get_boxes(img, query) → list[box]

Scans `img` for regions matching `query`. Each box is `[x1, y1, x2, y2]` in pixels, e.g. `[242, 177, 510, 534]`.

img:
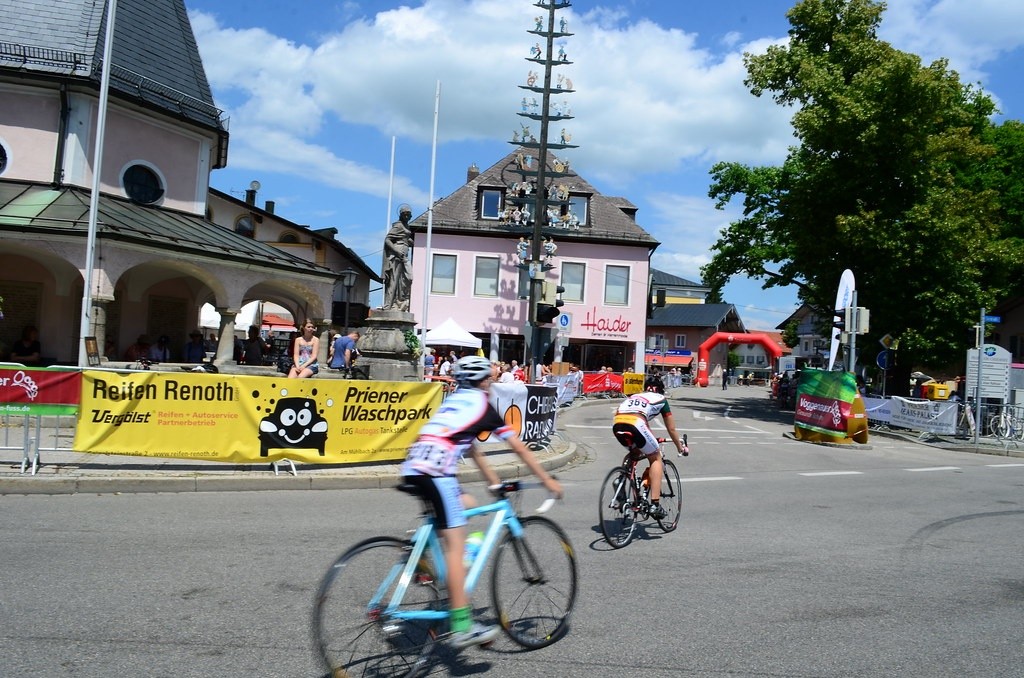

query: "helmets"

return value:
[644, 377, 664, 395]
[450, 355, 493, 381]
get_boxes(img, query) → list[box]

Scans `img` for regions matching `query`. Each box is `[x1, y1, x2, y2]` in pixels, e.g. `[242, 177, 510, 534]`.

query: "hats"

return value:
[189, 330, 203, 340]
[137, 334, 153, 346]
[159, 335, 169, 344]
[250, 325, 259, 331]
[334, 334, 342, 339]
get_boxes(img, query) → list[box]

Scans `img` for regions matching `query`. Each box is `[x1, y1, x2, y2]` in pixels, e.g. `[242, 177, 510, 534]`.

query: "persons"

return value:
[104, 335, 118, 361]
[183, 330, 243, 365]
[855, 375, 866, 397]
[598, 366, 613, 374]
[327, 330, 360, 379]
[425, 349, 579, 382]
[770, 372, 799, 411]
[379, 206, 415, 312]
[125, 334, 149, 363]
[266, 330, 275, 356]
[498, 0, 581, 264]
[288, 322, 320, 378]
[243, 326, 268, 365]
[612, 377, 684, 516]
[149, 335, 175, 363]
[10, 326, 41, 366]
[645, 368, 682, 389]
[722, 369, 727, 390]
[398, 355, 565, 649]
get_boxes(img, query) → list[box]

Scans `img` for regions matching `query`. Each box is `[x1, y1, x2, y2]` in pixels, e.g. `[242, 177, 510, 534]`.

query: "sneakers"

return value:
[404, 554, 439, 583]
[448, 621, 502, 649]
[648, 503, 668, 516]
[612, 478, 625, 502]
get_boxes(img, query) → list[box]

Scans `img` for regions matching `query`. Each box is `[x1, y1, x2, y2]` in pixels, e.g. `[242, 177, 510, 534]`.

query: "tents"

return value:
[415, 317, 482, 358]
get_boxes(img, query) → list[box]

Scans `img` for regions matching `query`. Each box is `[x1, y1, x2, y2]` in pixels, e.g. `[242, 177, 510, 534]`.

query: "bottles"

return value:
[465, 529, 484, 570]
[641, 467, 650, 487]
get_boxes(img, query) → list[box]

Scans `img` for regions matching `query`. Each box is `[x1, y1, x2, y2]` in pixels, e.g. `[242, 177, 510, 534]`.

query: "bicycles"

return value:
[957, 401, 998, 438]
[990, 403, 1024, 441]
[311, 474, 579, 678]
[599, 431, 687, 548]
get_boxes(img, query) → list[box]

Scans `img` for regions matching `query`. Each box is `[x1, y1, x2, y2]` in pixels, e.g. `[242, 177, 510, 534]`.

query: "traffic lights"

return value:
[537, 303, 560, 323]
[545, 282, 565, 307]
[833, 308, 850, 332]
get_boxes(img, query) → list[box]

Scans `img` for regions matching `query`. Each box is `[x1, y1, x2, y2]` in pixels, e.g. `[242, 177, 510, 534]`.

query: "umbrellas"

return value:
[262, 313, 294, 331]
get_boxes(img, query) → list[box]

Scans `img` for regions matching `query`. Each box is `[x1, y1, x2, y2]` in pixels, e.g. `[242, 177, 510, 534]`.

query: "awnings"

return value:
[645, 355, 694, 367]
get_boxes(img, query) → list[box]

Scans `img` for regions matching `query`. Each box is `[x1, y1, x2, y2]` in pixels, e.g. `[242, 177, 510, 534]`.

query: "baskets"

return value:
[353, 364, 370, 379]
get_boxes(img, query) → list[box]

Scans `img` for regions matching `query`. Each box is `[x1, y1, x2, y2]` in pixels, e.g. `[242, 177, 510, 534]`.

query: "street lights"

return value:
[340, 267, 359, 336]
